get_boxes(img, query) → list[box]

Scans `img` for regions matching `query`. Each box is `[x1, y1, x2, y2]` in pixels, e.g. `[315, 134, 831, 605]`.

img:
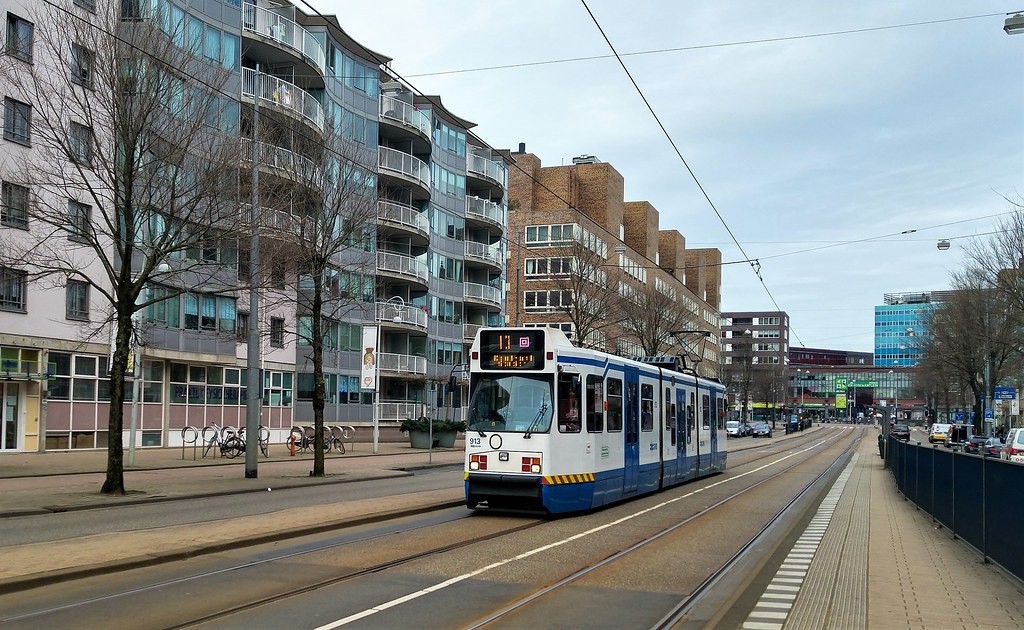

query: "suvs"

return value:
[999, 428, 1024, 463]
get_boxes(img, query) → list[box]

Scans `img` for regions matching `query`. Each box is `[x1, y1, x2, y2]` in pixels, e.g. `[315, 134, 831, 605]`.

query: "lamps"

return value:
[743, 328, 752, 334]
[936, 239, 951, 251]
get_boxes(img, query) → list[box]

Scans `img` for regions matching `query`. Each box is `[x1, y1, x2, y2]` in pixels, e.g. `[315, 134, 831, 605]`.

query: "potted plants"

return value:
[400, 416, 467, 448]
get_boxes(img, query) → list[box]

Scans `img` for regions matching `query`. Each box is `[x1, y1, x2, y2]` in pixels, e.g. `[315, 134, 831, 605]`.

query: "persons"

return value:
[995, 428, 998, 438]
[1000, 423, 1005, 443]
[858, 417, 861, 424]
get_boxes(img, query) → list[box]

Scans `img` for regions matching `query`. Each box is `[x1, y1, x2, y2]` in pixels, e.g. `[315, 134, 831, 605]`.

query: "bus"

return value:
[448, 326, 729, 515]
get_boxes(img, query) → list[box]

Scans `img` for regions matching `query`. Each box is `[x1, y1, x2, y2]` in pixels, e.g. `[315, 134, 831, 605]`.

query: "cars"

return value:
[726, 421, 753, 436]
[891, 424, 911, 442]
[961, 435, 1006, 458]
[928, 423, 953, 443]
[783, 415, 798, 430]
[751, 423, 772, 438]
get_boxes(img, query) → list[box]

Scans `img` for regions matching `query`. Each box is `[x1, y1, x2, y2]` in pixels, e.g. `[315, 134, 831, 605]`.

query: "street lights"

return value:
[797, 369, 810, 405]
[889, 360, 898, 408]
[726, 385, 735, 420]
[127, 232, 174, 468]
[752, 391, 759, 421]
[373, 296, 404, 453]
[776, 382, 784, 407]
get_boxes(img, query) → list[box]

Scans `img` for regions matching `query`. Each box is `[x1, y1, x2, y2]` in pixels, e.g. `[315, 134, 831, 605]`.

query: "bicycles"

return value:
[286, 426, 346, 454]
[203, 421, 271, 459]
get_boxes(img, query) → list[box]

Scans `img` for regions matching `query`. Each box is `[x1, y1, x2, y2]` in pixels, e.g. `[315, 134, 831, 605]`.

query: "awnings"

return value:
[788, 402, 825, 410]
[753, 402, 774, 408]
